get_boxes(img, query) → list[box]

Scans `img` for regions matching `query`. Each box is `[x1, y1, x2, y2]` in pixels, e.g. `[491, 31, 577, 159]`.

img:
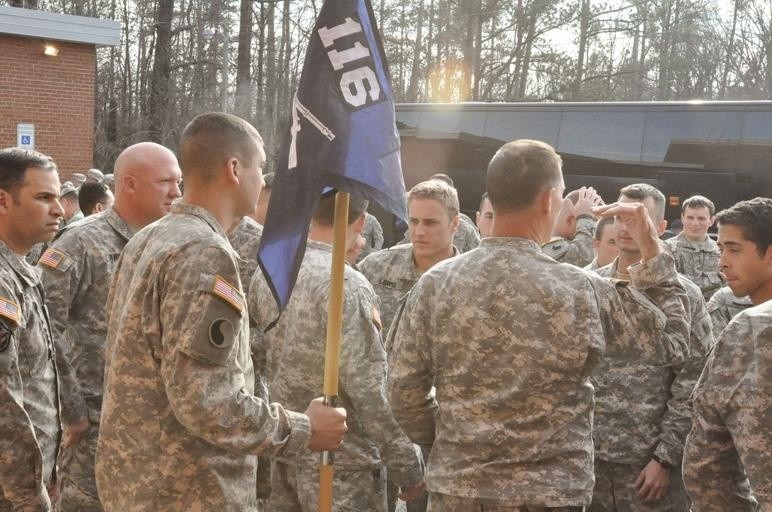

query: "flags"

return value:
[252, 1, 410, 333]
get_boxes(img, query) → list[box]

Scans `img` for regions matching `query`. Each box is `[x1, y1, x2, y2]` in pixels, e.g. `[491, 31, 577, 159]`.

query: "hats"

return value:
[60, 169, 115, 200]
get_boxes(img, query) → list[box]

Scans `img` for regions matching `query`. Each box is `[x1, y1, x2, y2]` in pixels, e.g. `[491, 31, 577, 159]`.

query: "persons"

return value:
[1, 109, 771, 511]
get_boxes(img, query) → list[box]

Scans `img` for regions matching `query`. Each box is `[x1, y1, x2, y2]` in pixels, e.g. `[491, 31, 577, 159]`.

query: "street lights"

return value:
[203, 27, 231, 114]
